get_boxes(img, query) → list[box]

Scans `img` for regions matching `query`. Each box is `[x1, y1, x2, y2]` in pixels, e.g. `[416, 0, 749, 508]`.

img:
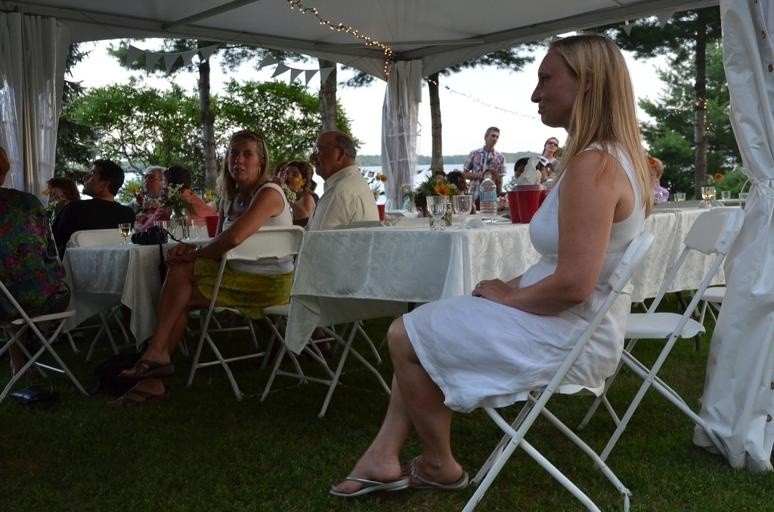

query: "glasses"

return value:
[548, 141, 558, 148]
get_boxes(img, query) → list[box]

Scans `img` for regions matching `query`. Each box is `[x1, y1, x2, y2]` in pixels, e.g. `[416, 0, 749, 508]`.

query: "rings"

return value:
[476, 283, 483, 288]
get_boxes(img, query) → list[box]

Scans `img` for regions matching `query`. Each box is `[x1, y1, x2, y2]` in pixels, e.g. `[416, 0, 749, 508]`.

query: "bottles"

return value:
[479, 173, 497, 223]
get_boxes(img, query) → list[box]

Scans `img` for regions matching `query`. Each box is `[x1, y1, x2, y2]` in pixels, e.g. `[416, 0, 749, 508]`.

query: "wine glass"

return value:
[190, 219, 203, 241]
[427, 196, 446, 229]
[118, 223, 131, 247]
[452, 195, 474, 230]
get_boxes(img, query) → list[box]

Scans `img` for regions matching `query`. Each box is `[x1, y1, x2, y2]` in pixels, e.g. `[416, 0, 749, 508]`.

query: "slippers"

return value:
[403, 454, 468, 490]
[119, 360, 175, 378]
[106, 387, 169, 408]
[330, 477, 409, 497]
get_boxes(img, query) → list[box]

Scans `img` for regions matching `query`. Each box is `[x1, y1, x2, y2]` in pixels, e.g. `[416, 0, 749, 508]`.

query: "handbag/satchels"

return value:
[96, 336, 152, 395]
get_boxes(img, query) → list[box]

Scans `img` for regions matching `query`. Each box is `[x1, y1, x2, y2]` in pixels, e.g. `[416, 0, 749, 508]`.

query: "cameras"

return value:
[131, 225, 169, 245]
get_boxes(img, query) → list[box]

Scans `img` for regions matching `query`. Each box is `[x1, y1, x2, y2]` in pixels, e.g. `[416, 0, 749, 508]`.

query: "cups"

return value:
[721, 191, 731, 200]
[700, 186, 716, 201]
[153, 220, 162, 227]
[205, 216, 220, 238]
[674, 192, 686, 201]
[162, 220, 176, 238]
[507, 185, 551, 223]
[738, 193, 751, 210]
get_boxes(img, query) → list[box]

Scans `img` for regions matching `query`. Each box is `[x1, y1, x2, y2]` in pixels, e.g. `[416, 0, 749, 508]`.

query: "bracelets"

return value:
[196, 245, 202, 257]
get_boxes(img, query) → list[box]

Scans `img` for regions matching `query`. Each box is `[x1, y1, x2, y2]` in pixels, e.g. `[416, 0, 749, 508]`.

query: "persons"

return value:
[304, 131, 380, 354]
[330, 34, 652, 498]
[0, 146, 71, 379]
[414, 127, 561, 217]
[107, 129, 294, 410]
[127, 165, 172, 224]
[51, 159, 136, 261]
[651, 157, 670, 204]
[47, 176, 81, 206]
[134, 166, 218, 232]
[275, 163, 288, 179]
[284, 161, 316, 227]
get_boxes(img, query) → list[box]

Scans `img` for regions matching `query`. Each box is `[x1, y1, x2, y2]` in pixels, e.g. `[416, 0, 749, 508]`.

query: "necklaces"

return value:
[236, 193, 253, 207]
